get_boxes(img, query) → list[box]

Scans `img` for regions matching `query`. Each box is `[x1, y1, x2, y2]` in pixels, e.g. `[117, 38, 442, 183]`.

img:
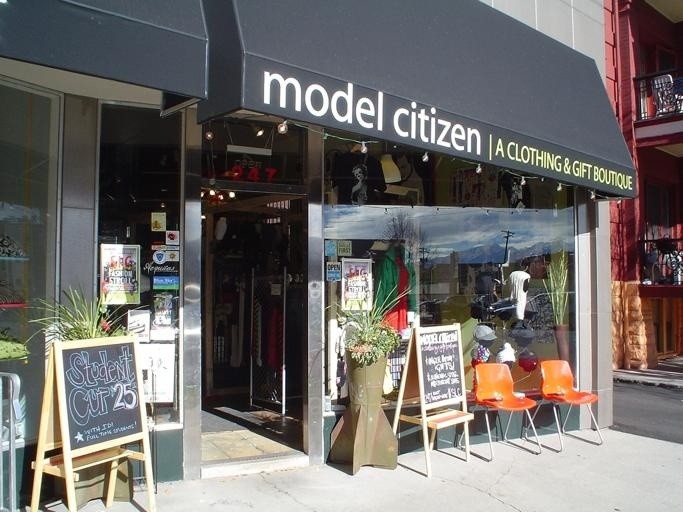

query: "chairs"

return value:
[460, 362, 542, 463]
[524, 359, 604, 453]
[650, 74, 683, 118]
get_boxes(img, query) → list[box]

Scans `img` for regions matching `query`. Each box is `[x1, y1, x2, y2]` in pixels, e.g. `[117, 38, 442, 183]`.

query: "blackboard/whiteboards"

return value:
[415, 323, 466, 410]
[53, 334, 148, 458]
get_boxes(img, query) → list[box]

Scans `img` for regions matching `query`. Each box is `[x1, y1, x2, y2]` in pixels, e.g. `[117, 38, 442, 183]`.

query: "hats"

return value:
[509, 319, 534, 338]
[519, 349, 536, 363]
[471, 345, 490, 368]
[496, 343, 517, 364]
[473, 324, 498, 342]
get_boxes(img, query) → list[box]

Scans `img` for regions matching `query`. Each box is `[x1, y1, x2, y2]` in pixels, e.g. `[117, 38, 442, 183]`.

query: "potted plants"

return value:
[26, 283, 149, 511]
[541, 252, 570, 361]
[331, 282, 409, 475]
